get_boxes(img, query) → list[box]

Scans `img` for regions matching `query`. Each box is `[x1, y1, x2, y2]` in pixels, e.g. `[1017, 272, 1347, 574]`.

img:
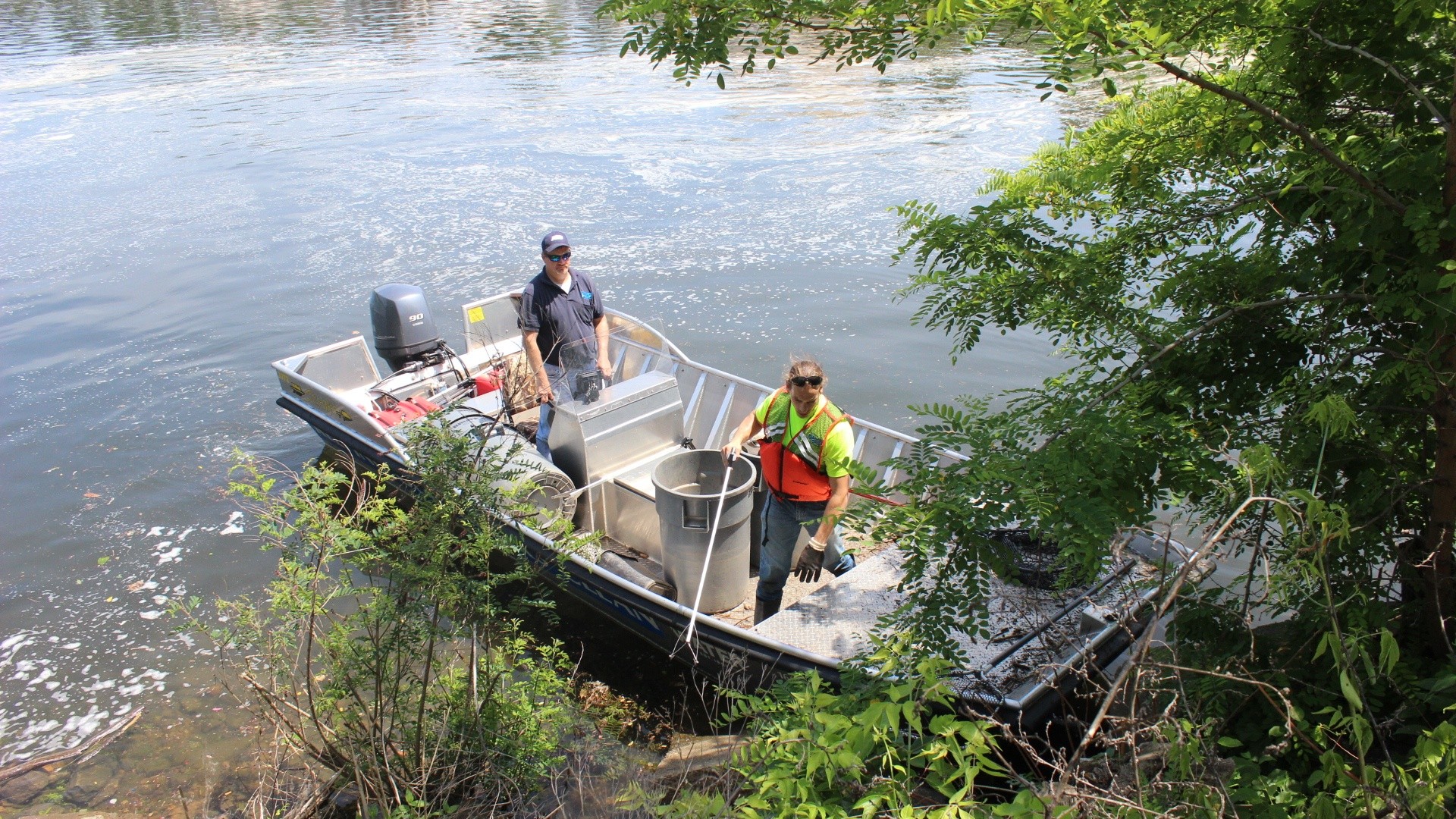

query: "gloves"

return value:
[794, 536, 827, 583]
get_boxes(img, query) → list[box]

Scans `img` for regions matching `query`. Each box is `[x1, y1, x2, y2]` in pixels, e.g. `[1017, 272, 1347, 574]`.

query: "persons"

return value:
[516, 231, 612, 464]
[720, 351, 858, 626]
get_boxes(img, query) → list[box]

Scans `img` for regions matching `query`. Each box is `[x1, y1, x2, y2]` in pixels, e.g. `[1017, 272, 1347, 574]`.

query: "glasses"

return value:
[789, 376, 823, 387]
[544, 251, 571, 262]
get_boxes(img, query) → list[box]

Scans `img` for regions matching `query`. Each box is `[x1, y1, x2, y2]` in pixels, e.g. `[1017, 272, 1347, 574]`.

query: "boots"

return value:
[754, 594, 781, 626]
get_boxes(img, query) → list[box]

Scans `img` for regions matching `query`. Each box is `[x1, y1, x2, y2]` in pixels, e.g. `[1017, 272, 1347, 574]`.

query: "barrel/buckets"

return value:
[650, 448, 757, 613]
[729, 425, 810, 570]
[371, 396, 578, 541]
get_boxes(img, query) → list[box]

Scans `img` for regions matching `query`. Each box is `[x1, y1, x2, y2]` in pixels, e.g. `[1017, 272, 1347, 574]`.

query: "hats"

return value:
[541, 232, 571, 252]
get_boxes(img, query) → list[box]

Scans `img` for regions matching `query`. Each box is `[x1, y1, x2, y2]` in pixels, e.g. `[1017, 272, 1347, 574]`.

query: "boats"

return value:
[272, 282, 1218, 745]
